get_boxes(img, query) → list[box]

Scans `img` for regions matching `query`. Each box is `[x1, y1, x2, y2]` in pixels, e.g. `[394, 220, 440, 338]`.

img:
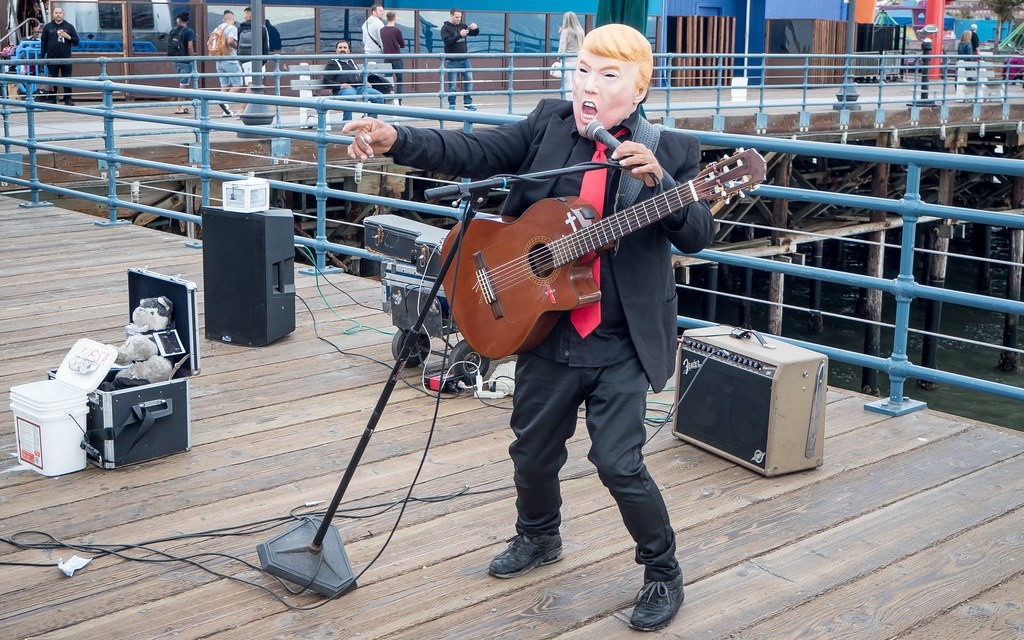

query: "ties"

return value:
[569, 127, 627, 339]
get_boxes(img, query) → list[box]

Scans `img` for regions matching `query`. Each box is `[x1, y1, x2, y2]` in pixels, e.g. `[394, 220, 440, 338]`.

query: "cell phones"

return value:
[466, 26, 471, 30]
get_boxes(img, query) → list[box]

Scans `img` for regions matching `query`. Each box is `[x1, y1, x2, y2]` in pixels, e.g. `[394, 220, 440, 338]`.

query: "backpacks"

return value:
[167, 27, 187, 56]
[237, 29, 252, 55]
[207, 26, 230, 56]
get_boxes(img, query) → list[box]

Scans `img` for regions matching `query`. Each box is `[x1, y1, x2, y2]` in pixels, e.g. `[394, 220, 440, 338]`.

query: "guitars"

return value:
[441, 146, 769, 358]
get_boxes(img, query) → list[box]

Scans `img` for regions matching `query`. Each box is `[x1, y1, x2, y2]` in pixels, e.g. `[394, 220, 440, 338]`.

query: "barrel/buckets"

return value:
[8, 382, 99, 475]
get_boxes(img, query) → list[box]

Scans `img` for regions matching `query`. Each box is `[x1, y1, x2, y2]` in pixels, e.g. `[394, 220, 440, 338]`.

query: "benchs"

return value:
[956, 59, 1006, 104]
[288, 61, 401, 133]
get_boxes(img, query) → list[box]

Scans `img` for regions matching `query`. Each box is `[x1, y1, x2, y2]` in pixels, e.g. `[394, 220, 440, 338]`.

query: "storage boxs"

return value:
[222, 179, 270, 214]
[46, 267, 201, 468]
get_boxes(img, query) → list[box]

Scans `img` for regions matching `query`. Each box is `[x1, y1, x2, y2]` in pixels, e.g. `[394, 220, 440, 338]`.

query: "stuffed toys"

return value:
[110, 294, 173, 365]
[113, 354, 171, 386]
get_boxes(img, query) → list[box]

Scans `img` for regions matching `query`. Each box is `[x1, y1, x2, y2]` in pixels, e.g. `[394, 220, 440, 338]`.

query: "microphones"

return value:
[584, 119, 646, 168]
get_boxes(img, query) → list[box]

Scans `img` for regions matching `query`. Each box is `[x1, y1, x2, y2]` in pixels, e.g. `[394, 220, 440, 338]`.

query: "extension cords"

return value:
[474, 390, 504, 399]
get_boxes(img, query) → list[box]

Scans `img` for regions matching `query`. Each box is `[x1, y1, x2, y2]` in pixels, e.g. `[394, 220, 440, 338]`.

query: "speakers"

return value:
[671, 326, 828, 477]
[201, 206, 296, 347]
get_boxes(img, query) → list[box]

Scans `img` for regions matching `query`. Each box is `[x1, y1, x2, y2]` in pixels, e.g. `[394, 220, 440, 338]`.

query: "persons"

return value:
[379, 11, 406, 106]
[341, 25, 715, 631]
[426, 25, 433, 54]
[41, 7, 79, 107]
[957, 31, 974, 81]
[213, 10, 244, 118]
[969, 24, 981, 69]
[236, 6, 269, 119]
[361, 4, 386, 77]
[265, 20, 282, 52]
[168, 12, 196, 114]
[556, 11, 585, 102]
[441, 7, 479, 111]
[323, 40, 386, 132]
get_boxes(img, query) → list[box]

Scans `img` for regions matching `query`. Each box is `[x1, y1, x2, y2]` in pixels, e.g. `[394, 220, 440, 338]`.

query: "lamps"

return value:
[916, 23, 939, 42]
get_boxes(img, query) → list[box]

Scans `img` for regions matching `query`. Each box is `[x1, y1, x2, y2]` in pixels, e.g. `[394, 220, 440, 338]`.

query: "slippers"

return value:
[175, 108, 189, 114]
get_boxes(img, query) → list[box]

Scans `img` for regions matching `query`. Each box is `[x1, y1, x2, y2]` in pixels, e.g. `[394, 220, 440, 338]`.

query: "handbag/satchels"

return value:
[550, 58, 568, 79]
[368, 75, 394, 93]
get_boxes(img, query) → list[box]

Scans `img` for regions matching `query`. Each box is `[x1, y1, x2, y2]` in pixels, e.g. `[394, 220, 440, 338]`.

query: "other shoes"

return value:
[223, 110, 233, 117]
[220, 104, 230, 114]
[64, 99, 75, 105]
[465, 105, 477, 110]
[448, 103, 456, 110]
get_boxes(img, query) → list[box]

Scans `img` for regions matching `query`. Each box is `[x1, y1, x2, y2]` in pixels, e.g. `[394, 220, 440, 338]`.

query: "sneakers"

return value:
[490, 532, 564, 579]
[629, 574, 684, 632]
